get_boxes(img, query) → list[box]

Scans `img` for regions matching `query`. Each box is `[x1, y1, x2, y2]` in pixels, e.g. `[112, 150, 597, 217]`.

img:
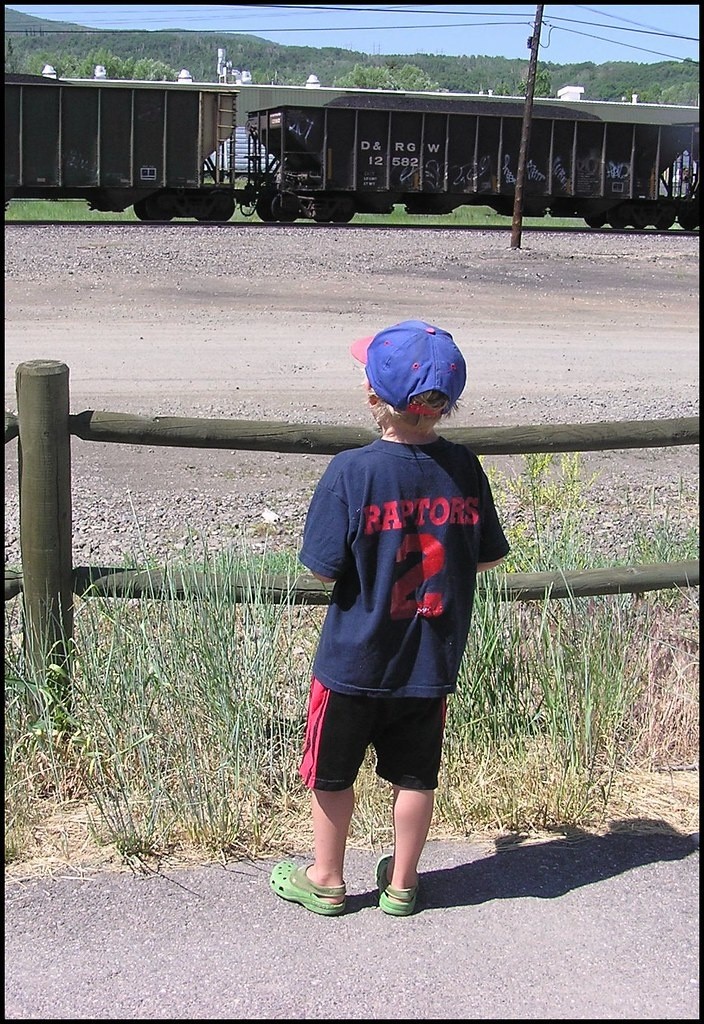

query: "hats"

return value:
[352, 320, 467, 415]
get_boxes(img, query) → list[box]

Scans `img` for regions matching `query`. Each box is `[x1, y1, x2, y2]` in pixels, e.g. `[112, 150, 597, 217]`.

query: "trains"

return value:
[3, 70, 699, 230]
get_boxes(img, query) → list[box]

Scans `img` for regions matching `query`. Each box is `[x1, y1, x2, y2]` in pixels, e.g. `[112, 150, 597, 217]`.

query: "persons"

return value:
[272, 322, 510, 915]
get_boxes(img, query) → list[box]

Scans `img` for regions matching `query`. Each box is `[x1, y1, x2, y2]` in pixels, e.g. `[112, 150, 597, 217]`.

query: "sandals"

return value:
[376, 852, 419, 915]
[270, 860, 347, 915]
